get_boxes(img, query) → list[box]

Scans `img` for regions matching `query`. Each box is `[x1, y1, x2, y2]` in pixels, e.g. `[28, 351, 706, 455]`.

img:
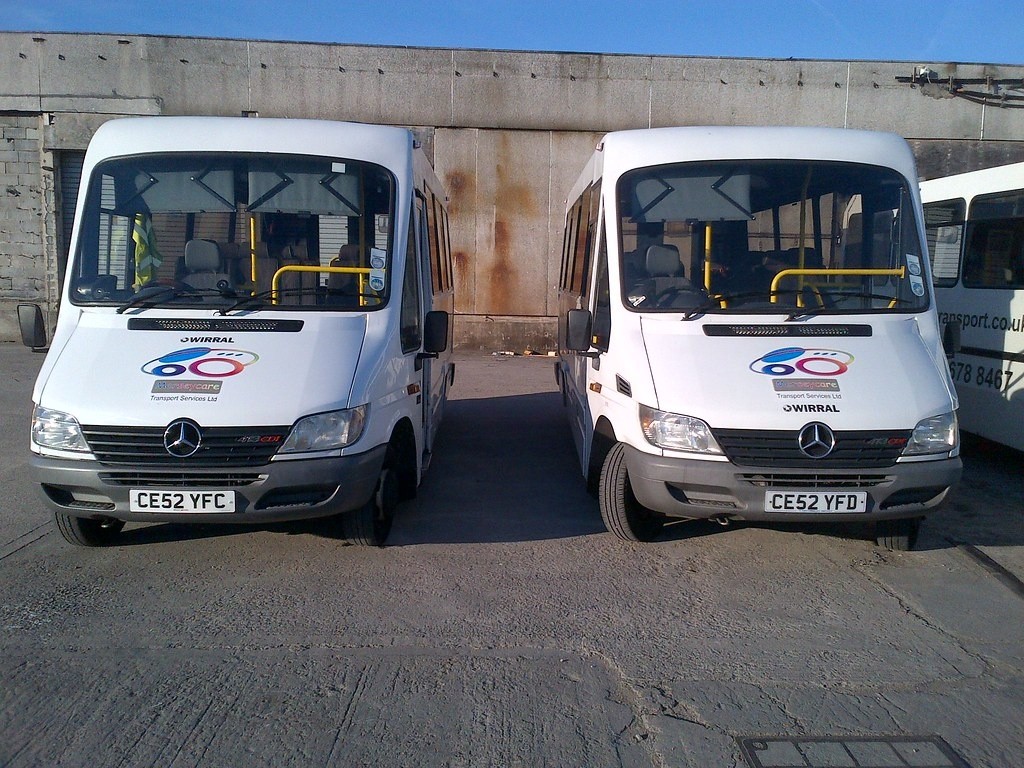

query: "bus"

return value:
[844, 161, 1023, 455]
[18, 115, 455, 546]
[551, 124, 963, 551]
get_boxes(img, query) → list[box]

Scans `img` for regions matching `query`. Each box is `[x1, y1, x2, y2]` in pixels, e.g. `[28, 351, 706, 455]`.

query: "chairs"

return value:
[727, 248, 827, 294]
[627, 244, 706, 307]
[325, 245, 370, 306]
[173, 238, 320, 304]
